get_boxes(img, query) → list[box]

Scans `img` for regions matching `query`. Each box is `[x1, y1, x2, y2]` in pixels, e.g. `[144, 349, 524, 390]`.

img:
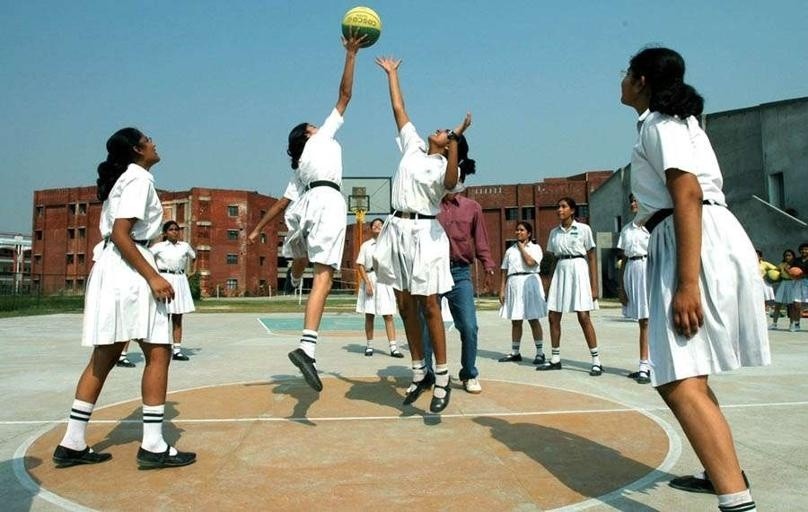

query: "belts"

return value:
[305, 181, 342, 193]
[627, 252, 648, 262]
[157, 268, 186, 276]
[104, 233, 153, 249]
[559, 252, 584, 259]
[642, 200, 718, 234]
[391, 206, 437, 221]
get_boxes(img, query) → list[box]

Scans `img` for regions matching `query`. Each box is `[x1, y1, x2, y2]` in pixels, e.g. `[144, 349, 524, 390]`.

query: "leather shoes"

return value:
[499, 353, 521, 362]
[628, 371, 650, 383]
[117, 357, 137, 368]
[289, 348, 322, 392]
[404, 370, 434, 405]
[534, 354, 546, 364]
[172, 350, 190, 361]
[669, 470, 749, 494]
[52, 444, 112, 464]
[590, 365, 602, 376]
[391, 350, 404, 358]
[137, 445, 197, 467]
[365, 346, 373, 356]
[430, 376, 450, 413]
[537, 361, 561, 370]
[285, 266, 298, 293]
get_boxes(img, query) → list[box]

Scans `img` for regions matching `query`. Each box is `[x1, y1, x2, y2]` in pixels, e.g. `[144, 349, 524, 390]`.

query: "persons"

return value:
[88, 240, 136, 369]
[245, 25, 373, 392]
[497, 221, 547, 363]
[755, 242, 807, 333]
[536, 197, 606, 375]
[354, 217, 405, 359]
[50, 127, 197, 469]
[617, 46, 776, 509]
[374, 51, 472, 412]
[147, 218, 198, 362]
[616, 192, 652, 381]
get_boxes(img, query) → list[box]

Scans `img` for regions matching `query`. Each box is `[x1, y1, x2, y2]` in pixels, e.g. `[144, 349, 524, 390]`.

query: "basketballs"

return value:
[341, 6, 383, 49]
[788, 266, 802, 276]
[767, 270, 780, 282]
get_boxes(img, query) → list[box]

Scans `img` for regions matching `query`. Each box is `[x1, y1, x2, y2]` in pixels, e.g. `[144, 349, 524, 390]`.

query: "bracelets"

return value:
[447, 130, 464, 144]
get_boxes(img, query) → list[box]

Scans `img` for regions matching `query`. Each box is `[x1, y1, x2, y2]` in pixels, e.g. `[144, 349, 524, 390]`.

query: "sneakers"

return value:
[788, 324, 800, 331]
[768, 323, 777, 329]
[462, 376, 482, 393]
